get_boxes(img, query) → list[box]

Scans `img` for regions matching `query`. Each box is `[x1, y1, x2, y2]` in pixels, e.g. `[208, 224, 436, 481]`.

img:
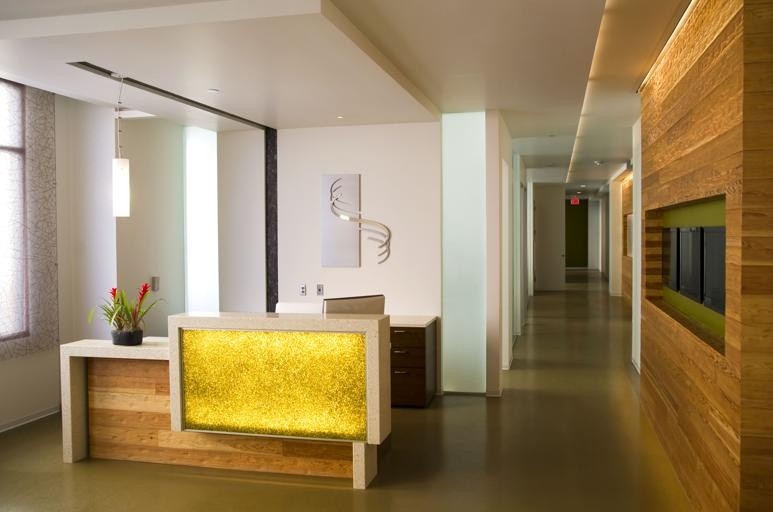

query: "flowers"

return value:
[84, 283, 167, 329]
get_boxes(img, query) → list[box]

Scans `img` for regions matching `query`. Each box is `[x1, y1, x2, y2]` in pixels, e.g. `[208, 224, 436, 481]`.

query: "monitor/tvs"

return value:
[322, 294, 385, 314]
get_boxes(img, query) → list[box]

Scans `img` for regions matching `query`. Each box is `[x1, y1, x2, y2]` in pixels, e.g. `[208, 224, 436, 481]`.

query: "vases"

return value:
[112, 329, 143, 346]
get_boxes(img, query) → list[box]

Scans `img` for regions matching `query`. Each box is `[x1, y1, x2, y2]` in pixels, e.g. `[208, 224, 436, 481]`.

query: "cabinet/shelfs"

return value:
[390, 327, 425, 409]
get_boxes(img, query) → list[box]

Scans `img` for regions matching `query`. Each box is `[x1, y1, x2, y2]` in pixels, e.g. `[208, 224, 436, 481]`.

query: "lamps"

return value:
[111, 72, 130, 218]
